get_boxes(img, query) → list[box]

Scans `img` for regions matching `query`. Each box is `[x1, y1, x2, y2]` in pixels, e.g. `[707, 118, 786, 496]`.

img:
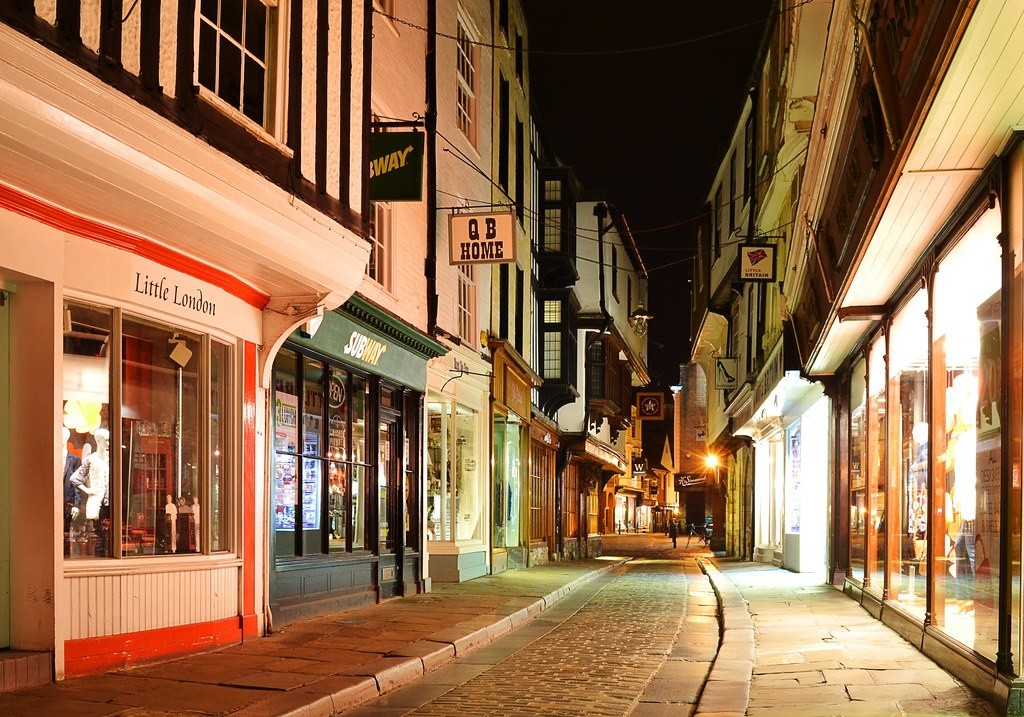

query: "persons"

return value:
[69, 429, 109, 557]
[178, 497, 192, 513]
[664, 519, 668, 535]
[669, 518, 676, 548]
[62, 427, 82, 561]
[165, 494, 177, 553]
[191, 497, 200, 552]
[677, 520, 681, 535]
[908, 373, 976, 617]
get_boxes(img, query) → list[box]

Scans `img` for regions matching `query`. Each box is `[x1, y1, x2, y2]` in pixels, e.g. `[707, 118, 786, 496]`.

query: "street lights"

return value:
[705, 452, 729, 558]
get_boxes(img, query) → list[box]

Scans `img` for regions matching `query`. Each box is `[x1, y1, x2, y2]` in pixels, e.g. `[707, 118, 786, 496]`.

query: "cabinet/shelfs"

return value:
[428, 413, 463, 544]
[272, 420, 364, 547]
[122, 434, 175, 555]
